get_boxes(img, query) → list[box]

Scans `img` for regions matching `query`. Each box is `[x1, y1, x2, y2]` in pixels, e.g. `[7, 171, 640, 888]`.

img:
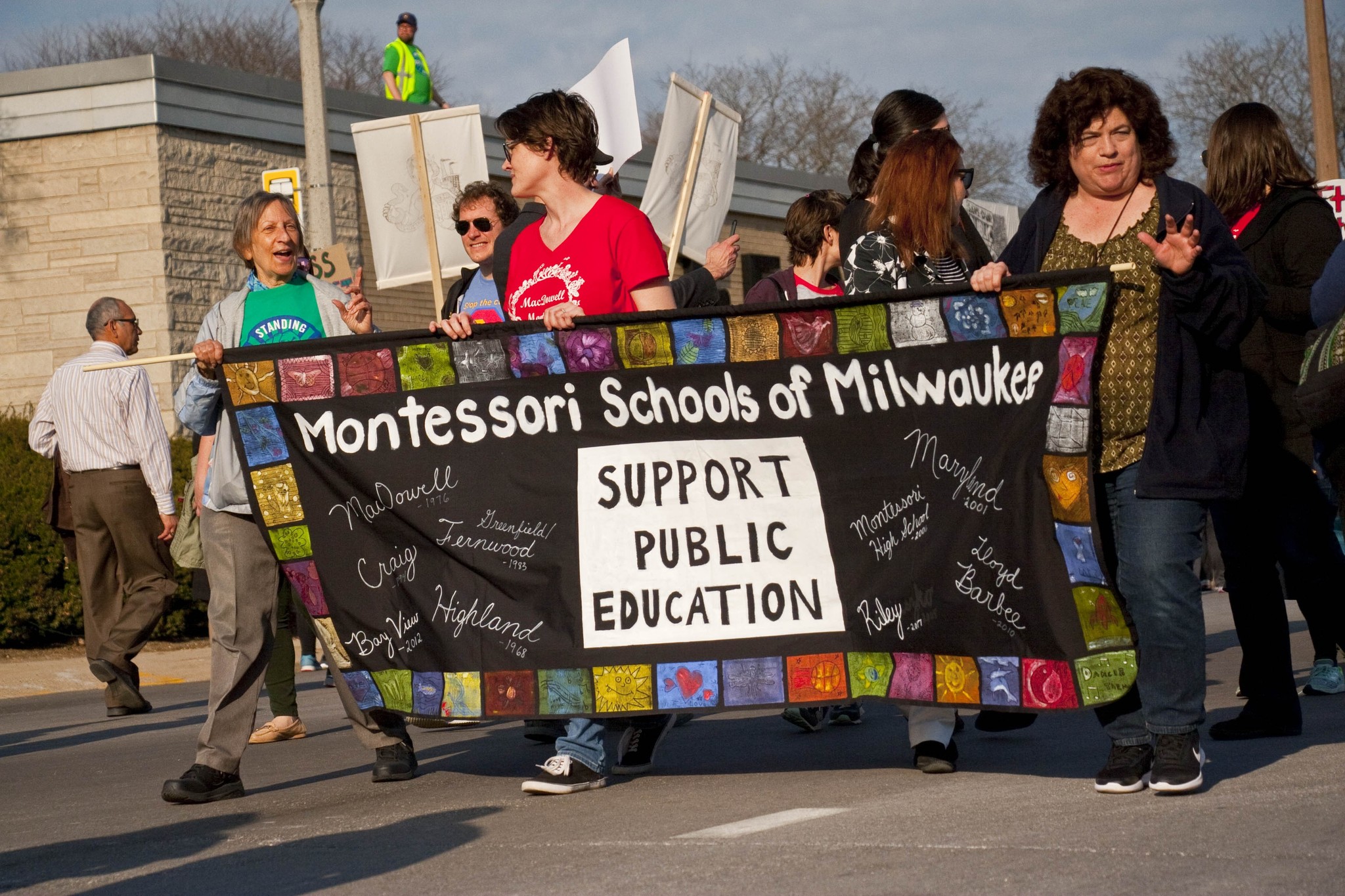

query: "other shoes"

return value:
[406, 715, 480, 728]
[250, 717, 307, 743]
[323, 672, 336, 688]
[319, 656, 329, 669]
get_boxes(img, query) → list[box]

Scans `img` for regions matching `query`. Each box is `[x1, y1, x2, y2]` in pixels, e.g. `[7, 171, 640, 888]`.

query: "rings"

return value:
[169, 532, 175, 536]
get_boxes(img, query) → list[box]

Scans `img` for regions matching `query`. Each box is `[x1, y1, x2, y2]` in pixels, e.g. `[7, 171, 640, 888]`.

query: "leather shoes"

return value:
[89, 660, 152, 717]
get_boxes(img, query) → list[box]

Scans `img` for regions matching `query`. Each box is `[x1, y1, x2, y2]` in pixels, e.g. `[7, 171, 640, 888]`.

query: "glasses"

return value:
[950, 167, 974, 190]
[502, 138, 525, 162]
[591, 168, 598, 182]
[399, 24, 414, 32]
[104, 318, 139, 330]
[296, 258, 312, 272]
[933, 124, 951, 132]
[455, 216, 502, 235]
[1200, 150, 1208, 169]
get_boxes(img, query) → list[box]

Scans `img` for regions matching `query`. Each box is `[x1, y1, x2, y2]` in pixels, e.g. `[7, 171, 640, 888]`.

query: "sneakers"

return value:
[161, 763, 244, 802]
[1201, 579, 1224, 592]
[522, 713, 694, 794]
[901, 660, 1344, 795]
[300, 655, 322, 671]
[372, 737, 415, 781]
[781, 702, 865, 729]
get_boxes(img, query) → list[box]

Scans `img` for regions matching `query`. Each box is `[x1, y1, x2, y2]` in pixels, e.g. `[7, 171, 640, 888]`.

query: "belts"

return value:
[104, 464, 141, 470]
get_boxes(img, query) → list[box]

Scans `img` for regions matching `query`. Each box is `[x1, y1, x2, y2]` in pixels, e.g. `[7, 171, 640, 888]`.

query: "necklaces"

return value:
[1095, 181, 1140, 260]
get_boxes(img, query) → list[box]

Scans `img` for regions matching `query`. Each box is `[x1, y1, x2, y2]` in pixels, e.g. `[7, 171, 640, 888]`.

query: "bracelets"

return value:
[440, 101, 445, 107]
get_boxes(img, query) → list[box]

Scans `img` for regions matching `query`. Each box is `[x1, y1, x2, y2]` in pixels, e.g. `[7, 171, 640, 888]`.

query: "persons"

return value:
[194, 434, 336, 742]
[28, 297, 177, 717]
[1204, 102, 1345, 737]
[430, 88, 675, 793]
[407, 180, 562, 734]
[746, 187, 862, 727]
[160, 189, 417, 802]
[972, 65, 1255, 792]
[591, 166, 743, 724]
[382, 12, 450, 108]
[840, 88, 1036, 771]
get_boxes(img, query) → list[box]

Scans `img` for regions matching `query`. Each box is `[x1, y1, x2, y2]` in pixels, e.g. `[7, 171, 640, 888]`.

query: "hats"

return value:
[592, 146, 613, 166]
[396, 12, 416, 29]
